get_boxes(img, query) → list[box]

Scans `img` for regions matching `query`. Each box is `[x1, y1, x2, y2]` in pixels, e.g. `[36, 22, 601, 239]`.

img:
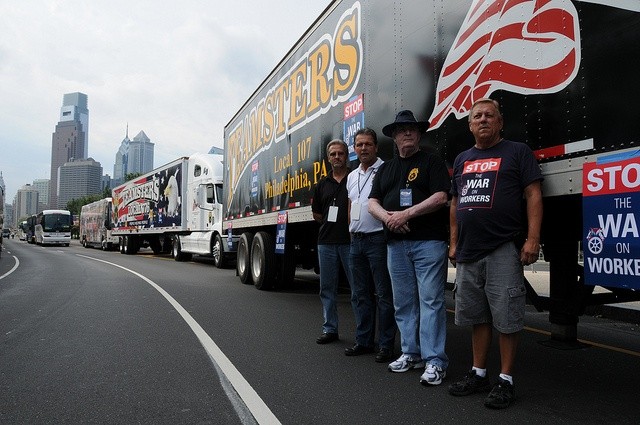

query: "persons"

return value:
[312, 139, 350, 345]
[344, 127, 398, 364]
[448, 98, 544, 410]
[368, 110, 452, 387]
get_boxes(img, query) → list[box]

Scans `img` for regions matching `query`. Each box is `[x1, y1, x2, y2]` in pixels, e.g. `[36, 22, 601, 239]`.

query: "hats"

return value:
[382, 110, 430, 137]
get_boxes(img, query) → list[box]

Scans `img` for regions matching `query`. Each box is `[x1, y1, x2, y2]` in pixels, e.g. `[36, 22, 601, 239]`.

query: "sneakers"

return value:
[448, 368, 489, 396]
[420, 362, 447, 385]
[484, 374, 516, 408]
[388, 353, 425, 372]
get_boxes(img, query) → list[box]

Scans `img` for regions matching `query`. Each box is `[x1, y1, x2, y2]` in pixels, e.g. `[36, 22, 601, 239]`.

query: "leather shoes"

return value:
[316, 332, 338, 343]
[374, 345, 393, 362]
[344, 344, 374, 355]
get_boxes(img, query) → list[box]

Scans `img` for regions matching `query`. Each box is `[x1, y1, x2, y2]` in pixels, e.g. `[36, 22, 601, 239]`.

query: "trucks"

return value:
[222, 0, 636, 290]
[112, 155, 224, 267]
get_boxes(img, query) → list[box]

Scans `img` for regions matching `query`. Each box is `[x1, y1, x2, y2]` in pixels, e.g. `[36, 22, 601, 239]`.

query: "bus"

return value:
[18, 221, 27, 241]
[27, 210, 70, 246]
[80, 198, 112, 250]
